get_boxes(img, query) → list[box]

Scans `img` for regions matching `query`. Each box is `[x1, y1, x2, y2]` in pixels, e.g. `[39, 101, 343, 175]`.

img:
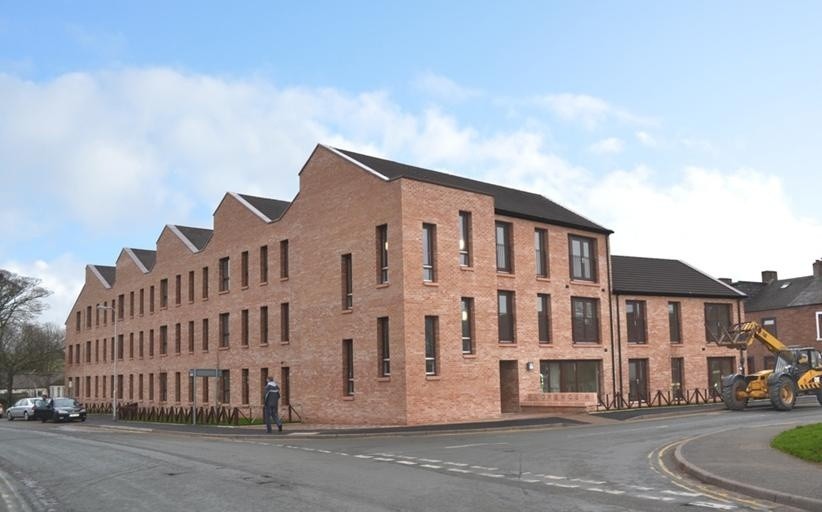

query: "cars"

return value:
[37, 397, 87, 422]
[6, 398, 42, 421]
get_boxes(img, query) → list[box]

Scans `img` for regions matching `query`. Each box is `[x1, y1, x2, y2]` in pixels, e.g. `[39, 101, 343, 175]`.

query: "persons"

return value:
[39, 395, 49, 423]
[264, 375, 282, 433]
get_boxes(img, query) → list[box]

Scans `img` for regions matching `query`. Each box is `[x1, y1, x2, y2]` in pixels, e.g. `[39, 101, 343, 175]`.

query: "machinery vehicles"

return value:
[704, 319, 822, 410]
